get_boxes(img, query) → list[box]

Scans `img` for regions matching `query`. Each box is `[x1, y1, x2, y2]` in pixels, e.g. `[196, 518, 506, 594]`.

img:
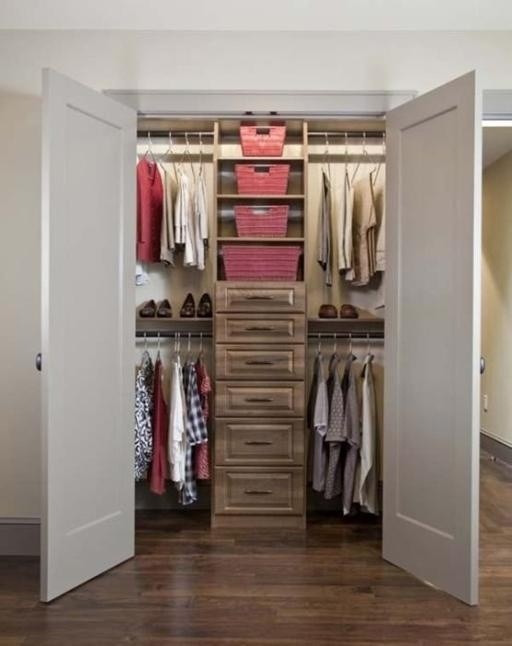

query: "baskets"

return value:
[220, 245, 303, 281]
[233, 205, 290, 237]
[234, 164, 292, 195]
[239, 125, 286, 157]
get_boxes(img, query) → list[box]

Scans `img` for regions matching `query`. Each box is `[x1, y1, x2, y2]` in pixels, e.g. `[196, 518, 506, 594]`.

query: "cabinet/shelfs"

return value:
[34, 63, 487, 614]
[211, 119, 307, 534]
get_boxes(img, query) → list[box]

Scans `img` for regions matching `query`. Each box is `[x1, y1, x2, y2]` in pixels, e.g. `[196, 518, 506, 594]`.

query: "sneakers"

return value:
[340, 303, 359, 319]
[318, 304, 337, 319]
[157, 299, 173, 318]
[139, 300, 157, 318]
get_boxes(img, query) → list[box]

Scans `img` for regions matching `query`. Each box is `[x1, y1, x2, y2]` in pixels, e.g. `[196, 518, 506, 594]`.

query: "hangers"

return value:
[317, 122, 386, 178]
[142, 128, 205, 180]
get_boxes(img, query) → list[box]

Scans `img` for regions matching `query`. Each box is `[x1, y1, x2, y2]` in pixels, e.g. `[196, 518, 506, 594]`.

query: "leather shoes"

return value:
[180, 293, 195, 317]
[197, 293, 212, 318]
